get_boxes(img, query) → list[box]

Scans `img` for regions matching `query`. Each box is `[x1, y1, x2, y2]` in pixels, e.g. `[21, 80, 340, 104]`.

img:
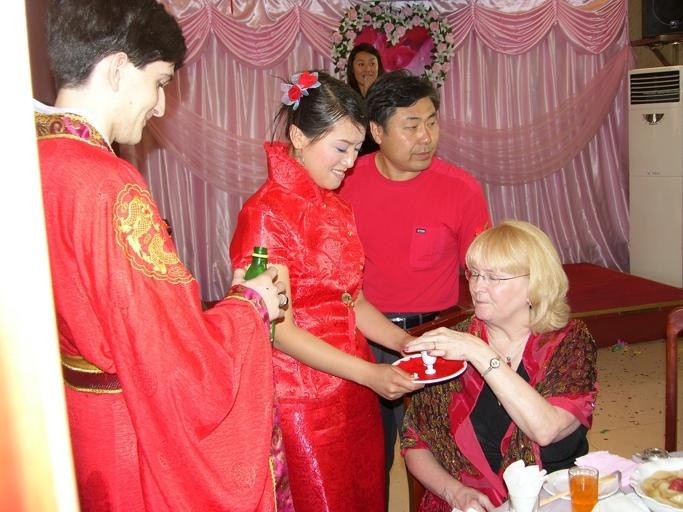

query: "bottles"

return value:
[240, 244, 276, 347]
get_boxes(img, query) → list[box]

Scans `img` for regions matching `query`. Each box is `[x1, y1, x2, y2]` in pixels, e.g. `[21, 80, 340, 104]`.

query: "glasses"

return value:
[464, 271, 530, 287]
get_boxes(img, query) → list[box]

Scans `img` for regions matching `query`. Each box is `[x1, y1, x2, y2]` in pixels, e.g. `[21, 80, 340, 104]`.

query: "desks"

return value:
[440, 262, 683, 452]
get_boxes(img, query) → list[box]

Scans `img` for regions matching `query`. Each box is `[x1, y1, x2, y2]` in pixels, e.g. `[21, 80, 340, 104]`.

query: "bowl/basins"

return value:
[630, 457, 682, 512]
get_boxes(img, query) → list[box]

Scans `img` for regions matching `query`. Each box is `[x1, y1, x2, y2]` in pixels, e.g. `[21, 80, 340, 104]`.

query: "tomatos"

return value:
[670, 477, 682, 492]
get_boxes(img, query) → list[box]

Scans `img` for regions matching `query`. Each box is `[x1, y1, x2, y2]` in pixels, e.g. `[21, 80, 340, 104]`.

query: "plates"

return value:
[542, 468, 619, 501]
[390, 353, 467, 384]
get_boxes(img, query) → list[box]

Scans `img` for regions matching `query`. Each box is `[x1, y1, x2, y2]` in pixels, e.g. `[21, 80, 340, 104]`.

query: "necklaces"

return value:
[487, 330, 530, 368]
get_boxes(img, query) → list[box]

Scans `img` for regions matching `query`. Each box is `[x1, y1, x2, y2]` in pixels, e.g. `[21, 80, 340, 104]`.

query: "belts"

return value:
[385, 311, 443, 330]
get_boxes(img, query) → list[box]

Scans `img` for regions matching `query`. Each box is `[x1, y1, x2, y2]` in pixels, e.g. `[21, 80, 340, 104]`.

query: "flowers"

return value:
[280, 70, 322, 111]
[332, 2, 455, 89]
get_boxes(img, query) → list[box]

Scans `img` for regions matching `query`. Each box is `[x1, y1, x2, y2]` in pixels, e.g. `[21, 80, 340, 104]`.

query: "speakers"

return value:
[641, 0, 683, 38]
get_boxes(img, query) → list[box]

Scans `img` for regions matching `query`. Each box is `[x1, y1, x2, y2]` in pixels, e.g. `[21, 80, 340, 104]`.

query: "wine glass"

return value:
[420, 351, 437, 377]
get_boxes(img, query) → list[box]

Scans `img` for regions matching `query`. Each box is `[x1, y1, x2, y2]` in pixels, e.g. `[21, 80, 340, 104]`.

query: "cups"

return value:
[507, 495, 539, 512]
[566, 465, 598, 512]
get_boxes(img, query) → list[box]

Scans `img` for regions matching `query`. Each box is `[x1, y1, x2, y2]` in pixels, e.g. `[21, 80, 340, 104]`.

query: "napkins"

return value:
[503, 459, 550, 512]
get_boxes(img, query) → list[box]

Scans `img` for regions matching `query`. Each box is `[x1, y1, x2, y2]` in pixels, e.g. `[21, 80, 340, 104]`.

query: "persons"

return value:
[228, 70, 419, 512]
[32, 1, 289, 512]
[346, 43, 384, 156]
[400, 217, 600, 512]
[334, 70, 492, 511]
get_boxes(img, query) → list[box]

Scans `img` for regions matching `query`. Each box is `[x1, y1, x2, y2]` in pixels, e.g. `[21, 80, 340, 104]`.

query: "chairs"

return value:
[403, 308, 475, 512]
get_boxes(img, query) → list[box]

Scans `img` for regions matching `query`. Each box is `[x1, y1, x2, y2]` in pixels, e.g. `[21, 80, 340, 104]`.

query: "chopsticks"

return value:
[540, 472, 615, 508]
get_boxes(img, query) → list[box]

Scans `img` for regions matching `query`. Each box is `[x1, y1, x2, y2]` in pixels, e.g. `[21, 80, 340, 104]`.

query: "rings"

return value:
[279, 293, 288, 308]
[433, 341, 435, 350]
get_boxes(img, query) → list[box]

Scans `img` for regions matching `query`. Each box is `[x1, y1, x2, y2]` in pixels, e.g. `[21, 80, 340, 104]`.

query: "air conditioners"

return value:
[627, 66, 683, 287]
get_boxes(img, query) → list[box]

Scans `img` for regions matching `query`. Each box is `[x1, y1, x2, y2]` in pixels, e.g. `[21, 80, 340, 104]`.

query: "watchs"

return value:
[480, 354, 501, 378]
